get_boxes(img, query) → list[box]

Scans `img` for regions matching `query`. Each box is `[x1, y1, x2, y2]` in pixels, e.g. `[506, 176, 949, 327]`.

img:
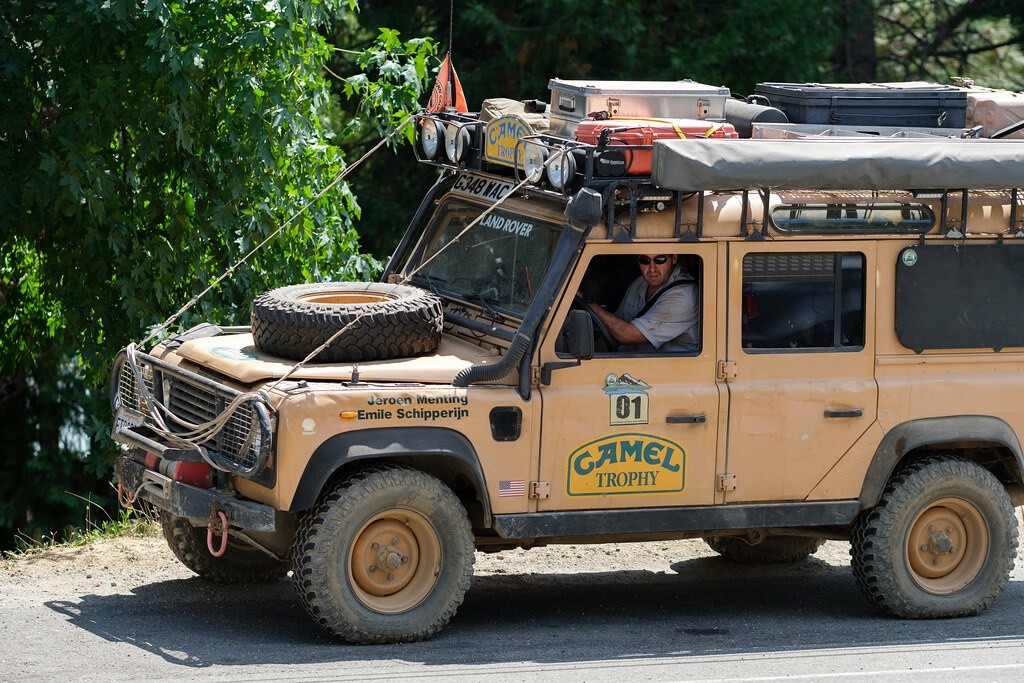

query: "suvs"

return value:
[108, 114, 1024, 647]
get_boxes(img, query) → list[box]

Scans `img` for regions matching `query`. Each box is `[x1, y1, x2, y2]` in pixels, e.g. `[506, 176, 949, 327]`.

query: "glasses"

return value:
[637, 254, 672, 265]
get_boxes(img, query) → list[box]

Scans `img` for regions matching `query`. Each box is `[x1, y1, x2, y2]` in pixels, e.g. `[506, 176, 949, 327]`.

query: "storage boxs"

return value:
[754, 81, 968, 129]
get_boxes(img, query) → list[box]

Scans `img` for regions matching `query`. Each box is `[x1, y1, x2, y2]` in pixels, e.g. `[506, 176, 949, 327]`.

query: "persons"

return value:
[576, 254, 702, 353]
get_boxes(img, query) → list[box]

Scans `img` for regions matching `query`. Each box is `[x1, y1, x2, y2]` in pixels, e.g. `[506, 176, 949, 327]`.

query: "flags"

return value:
[419, 53, 468, 127]
